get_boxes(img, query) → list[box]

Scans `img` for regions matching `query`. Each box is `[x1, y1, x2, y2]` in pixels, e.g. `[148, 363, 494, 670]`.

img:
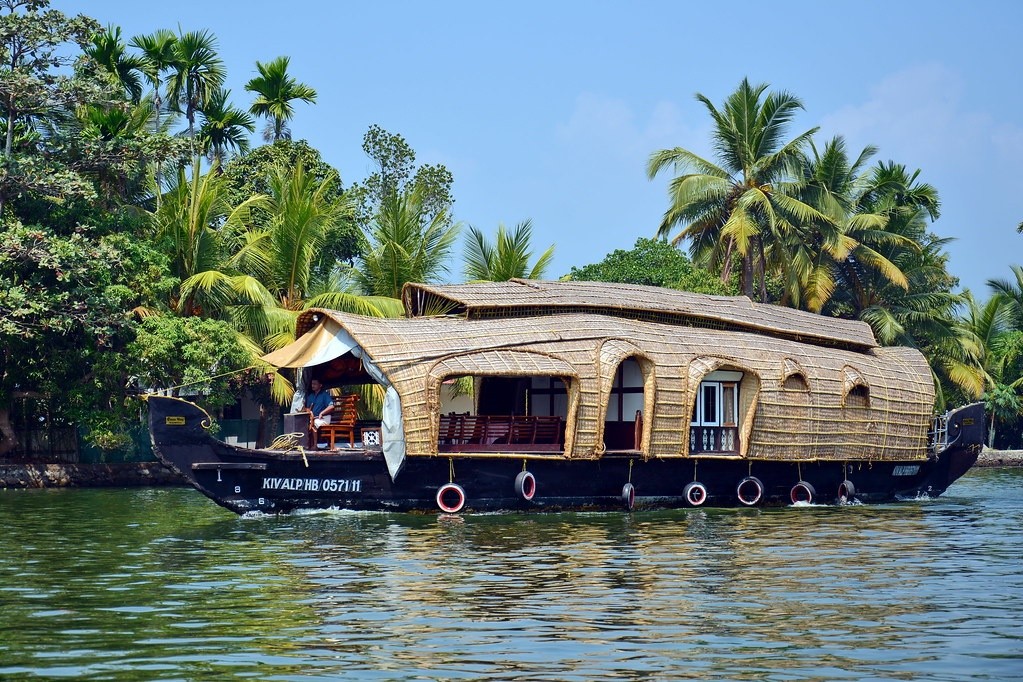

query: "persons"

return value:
[305, 377, 334, 451]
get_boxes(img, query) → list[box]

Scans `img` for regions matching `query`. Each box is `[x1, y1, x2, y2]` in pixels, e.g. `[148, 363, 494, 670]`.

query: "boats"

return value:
[147, 277, 987, 517]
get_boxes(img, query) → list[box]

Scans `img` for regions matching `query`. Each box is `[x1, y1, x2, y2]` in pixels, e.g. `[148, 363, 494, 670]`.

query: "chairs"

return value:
[439, 410, 563, 452]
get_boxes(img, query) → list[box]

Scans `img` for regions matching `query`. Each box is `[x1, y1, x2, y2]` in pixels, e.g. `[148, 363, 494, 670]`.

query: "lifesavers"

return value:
[682, 482, 707, 506]
[436, 483, 465, 514]
[790, 481, 816, 505]
[515, 470, 536, 501]
[621, 483, 634, 511]
[735, 476, 765, 506]
[838, 480, 855, 503]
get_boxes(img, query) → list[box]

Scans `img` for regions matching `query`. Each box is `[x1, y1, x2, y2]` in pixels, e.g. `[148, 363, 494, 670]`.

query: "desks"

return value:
[479, 423, 519, 445]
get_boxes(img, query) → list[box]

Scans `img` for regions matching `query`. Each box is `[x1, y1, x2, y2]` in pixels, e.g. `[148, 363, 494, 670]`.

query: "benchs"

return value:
[311, 394, 360, 451]
